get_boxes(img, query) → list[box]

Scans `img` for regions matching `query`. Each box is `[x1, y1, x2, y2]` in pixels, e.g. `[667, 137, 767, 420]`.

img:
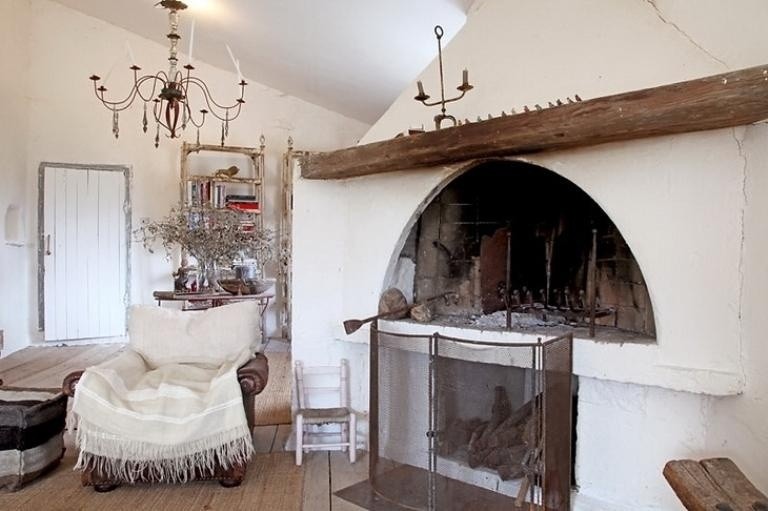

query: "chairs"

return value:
[61, 301, 267, 487]
[293, 360, 357, 466]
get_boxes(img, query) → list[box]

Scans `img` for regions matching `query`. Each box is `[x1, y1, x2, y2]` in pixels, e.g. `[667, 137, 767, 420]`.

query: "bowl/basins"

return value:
[218, 278, 277, 294]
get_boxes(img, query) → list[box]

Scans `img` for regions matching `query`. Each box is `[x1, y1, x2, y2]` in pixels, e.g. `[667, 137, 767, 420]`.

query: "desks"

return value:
[154, 288, 275, 343]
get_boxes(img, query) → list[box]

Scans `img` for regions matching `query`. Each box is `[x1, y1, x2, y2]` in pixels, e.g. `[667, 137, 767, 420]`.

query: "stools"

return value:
[663, 458, 768, 511]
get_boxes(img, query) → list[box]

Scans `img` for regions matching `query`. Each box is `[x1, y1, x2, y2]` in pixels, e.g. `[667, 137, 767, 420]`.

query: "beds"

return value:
[1, 346, 131, 493]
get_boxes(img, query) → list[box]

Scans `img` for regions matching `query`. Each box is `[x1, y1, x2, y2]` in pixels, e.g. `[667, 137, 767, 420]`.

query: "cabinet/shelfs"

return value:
[179, 141, 265, 279]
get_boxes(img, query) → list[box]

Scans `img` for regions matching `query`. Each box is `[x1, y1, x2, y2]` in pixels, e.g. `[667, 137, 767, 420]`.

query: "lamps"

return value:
[89, 0, 247, 153]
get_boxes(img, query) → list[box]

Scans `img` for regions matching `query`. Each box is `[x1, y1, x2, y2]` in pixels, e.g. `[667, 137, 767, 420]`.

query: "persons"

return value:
[172, 259, 197, 290]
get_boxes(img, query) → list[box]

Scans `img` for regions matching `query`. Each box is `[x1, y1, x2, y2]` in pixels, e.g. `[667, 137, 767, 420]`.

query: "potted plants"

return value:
[133, 202, 279, 297]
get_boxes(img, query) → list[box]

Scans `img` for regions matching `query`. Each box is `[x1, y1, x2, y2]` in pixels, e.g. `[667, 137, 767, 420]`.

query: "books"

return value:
[187, 179, 259, 234]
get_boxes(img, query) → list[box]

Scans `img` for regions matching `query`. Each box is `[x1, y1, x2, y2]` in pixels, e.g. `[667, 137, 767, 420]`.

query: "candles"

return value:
[463, 67, 468, 84]
[417, 81, 424, 96]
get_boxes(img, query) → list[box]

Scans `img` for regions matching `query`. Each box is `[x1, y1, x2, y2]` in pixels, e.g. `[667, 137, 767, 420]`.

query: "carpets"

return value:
[1, 344, 292, 427]
[1, 451, 330, 511]
